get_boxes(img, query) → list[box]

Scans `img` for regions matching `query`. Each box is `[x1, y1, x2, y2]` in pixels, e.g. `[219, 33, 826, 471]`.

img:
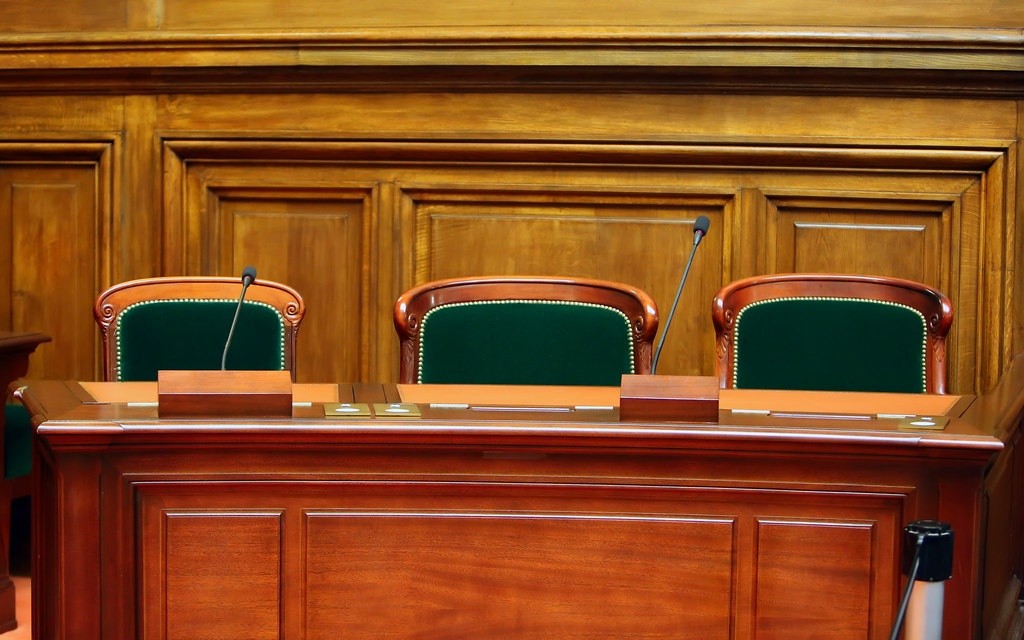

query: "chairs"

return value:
[394, 273, 661, 387]
[91, 276, 306, 380]
[711, 274, 955, 395]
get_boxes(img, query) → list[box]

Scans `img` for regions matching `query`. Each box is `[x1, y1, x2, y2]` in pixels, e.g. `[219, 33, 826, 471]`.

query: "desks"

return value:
[40, 403, 1005, 640]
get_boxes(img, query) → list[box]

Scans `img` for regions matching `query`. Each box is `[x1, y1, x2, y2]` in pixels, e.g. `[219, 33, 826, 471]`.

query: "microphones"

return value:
[220, 266, 258, 370]
[650, 216, 711, 375]
